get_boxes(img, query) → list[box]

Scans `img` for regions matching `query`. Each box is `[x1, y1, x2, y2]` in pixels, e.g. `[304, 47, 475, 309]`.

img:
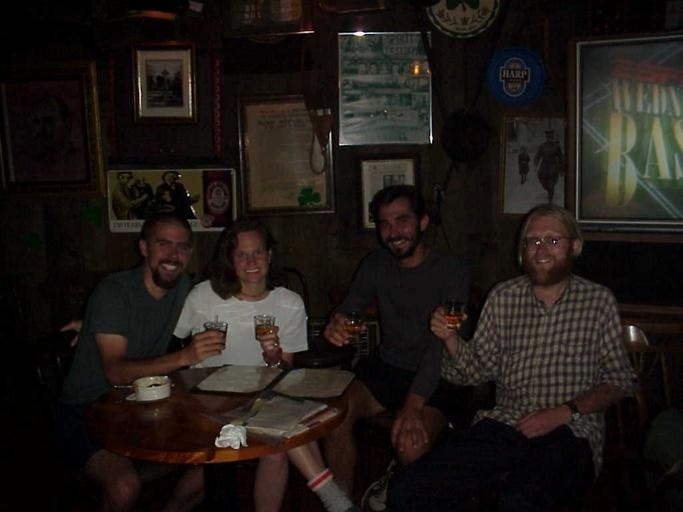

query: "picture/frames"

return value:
[105, 163, 242, 244]
[331, 28, 442, 153]
[355, 153, 421, 232]
[564, 31, 682, 247]
[231, 92, 336, 219]
[492, 107, 565, 223]
[0, 59, 106, 201]
[131, 40, 199, 128]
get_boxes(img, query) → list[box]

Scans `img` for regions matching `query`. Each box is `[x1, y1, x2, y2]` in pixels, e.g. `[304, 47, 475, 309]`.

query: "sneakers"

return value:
[361, 458, 396, 511]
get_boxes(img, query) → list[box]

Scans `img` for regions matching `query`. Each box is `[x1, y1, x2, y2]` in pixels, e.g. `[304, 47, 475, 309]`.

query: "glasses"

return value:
[521, 236, 567, 249]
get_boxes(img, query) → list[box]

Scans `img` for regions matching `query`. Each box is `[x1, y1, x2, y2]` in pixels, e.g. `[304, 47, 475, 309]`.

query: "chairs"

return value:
[615, 317, 682, 451]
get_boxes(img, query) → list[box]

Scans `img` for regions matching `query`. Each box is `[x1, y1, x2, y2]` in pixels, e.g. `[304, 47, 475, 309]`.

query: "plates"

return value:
[192, 365, 285, 397]
[273, 368, 358, 400]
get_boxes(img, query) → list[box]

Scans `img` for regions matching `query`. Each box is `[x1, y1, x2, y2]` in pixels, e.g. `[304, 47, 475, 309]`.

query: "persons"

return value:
[113, 170, 195, 216]
[535, 128, 564, 204]
[53, 211, 224, 506]
[360, 205, 641, 507]
[513, 142, 533, 186]
[60, 218, 353, 506]
[324, 184, 465, 464]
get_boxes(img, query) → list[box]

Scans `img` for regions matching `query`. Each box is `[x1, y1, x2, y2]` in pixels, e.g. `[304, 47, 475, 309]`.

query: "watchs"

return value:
[261, 359, 286, 368]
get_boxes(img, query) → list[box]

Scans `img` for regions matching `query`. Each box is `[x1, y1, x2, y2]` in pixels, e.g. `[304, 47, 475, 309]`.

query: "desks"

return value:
[84, 370, 348, 511]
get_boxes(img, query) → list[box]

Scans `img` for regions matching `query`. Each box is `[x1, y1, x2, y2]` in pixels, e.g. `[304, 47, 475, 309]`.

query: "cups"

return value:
[439, 296, 467, 329]
[253, 314, 274, 343]
[132, 375, 171, 402]
[203, 320, 228, 349]
[342, 304, 364, 333]
